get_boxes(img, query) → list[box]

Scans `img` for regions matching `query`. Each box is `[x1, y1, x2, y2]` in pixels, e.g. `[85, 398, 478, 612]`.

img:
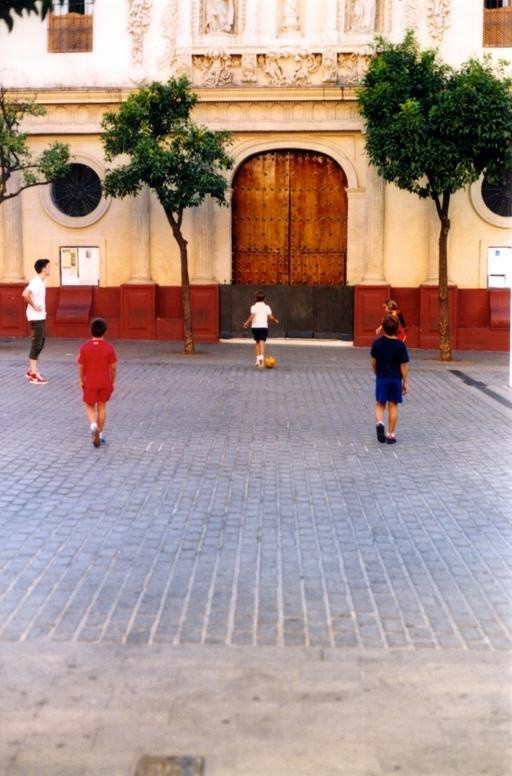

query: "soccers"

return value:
[265, 354, 276, 368]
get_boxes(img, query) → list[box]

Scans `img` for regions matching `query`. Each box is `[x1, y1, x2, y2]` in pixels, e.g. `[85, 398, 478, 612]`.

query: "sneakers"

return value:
[25, 370, 48, 384]
[92, 424, 105, 446]
[376, 423, 386, 443]
[387, 433, 397, 443]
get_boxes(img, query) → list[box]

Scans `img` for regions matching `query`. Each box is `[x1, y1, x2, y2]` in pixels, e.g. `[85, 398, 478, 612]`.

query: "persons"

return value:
[370, 314, 409, 444]
[79, 320, 116, 447]
[22, 260, 53, 385]
[243, 291, 279, 370]
[376, 300, 409, 349]
